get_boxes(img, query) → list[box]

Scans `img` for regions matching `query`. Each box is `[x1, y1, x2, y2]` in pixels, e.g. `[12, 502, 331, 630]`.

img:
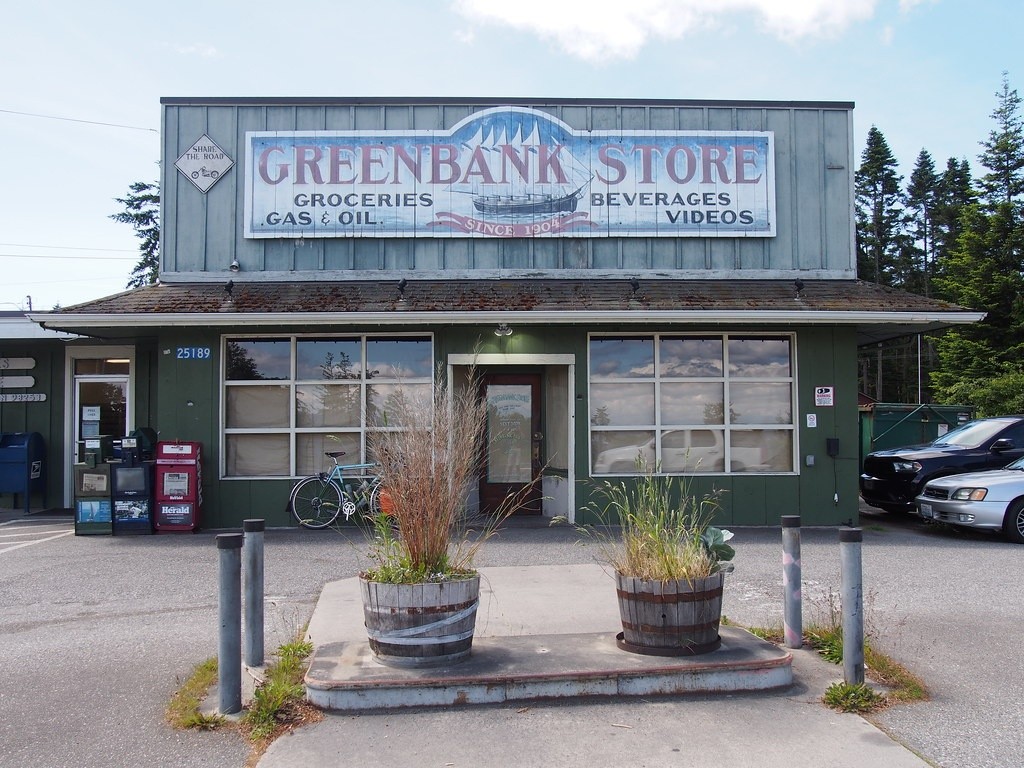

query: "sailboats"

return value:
[444, 120, 595, 215]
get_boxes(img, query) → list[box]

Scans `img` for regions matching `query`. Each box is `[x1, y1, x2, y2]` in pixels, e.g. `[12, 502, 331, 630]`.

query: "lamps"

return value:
[629, 276, 639, 294]
[395, 277, 409, 300]
[228, 259, 242, 273]
[495, 324, 514, 337]
[794, 277, 805, 299]
[223, 280, 236, 300]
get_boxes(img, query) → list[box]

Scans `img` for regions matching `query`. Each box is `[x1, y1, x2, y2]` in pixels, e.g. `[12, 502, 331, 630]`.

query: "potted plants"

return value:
[327, 334, 547, 669]
[544, 446, 737, 660]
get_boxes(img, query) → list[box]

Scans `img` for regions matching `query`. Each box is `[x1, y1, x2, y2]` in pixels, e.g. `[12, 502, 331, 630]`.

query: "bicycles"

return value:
[288, 449, 435, 532]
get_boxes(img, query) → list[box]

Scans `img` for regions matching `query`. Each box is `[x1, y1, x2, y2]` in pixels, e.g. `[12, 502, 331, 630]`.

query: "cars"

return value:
[861, 412, 1024, 509]
[913, 454, 1024, 545]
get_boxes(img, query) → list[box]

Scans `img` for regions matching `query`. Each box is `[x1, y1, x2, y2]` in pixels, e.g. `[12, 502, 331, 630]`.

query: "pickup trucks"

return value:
[594, 427, 763, 474]
